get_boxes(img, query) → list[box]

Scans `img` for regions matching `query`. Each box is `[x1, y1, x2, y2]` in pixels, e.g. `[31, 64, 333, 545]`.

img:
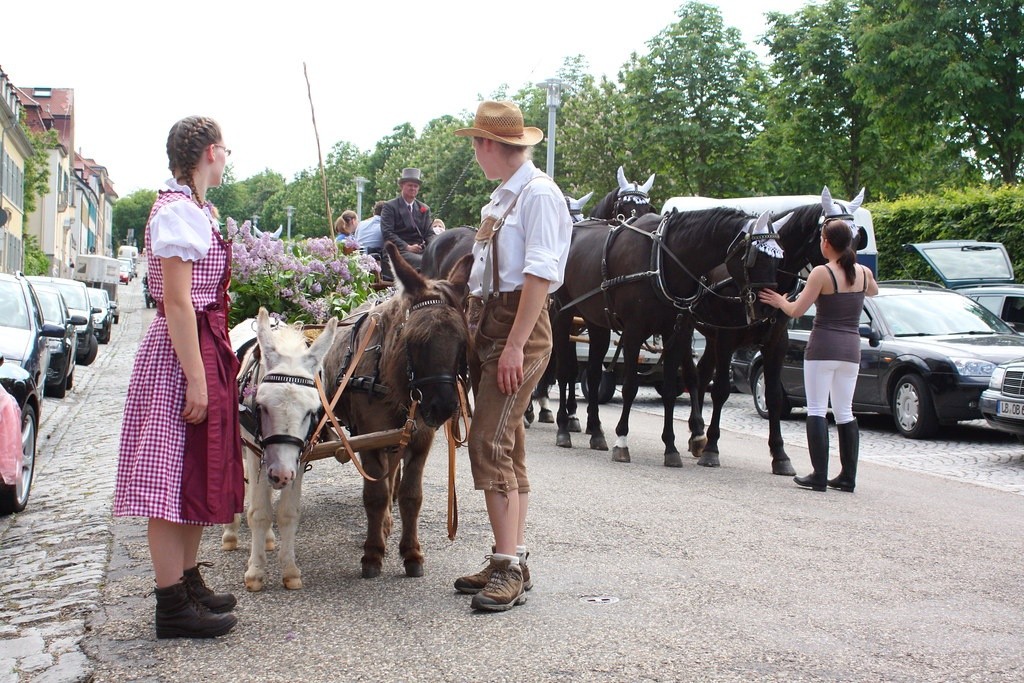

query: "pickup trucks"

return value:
[0, 270, 64, 419]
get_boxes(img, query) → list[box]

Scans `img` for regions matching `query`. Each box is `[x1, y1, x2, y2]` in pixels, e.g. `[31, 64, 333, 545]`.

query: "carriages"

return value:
[358, 165, 865, 478]
[215, 239, 475, 592]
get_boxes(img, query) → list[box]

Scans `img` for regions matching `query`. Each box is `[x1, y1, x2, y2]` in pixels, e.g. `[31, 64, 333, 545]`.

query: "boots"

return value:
[826, 418, 860, 494]
[793, 414, 829, 491]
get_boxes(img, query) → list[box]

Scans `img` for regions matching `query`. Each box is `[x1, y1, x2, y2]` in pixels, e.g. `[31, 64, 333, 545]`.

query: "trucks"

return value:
[570, 318, 733, 403]
[117, 245, 139, 265]
[70, 254, 120, 301]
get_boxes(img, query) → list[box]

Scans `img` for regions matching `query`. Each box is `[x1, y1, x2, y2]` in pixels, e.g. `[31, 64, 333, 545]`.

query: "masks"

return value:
[434, 227, 444, 235]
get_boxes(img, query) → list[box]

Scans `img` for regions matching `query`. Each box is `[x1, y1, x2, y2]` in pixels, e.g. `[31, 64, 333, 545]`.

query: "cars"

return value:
[0, 353, 40, 512]
[25, 276, 87, 399]
[89, 289, 118, 345]
[979, 356, 1024, 436]
[902, 239, 1024, 334]
[117, 259, 137, 284]
[54, 278, 102, 366]
[730, 279, 1024, 439]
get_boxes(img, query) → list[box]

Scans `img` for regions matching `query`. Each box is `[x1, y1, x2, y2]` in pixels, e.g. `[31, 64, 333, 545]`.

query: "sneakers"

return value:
[153, 578, 239, 639]
[183, 563, 237, 611]
[469, 556, 528, 610]
[454, 547, 534, 593]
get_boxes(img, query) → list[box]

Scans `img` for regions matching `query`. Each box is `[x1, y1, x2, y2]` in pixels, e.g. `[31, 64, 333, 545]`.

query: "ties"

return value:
[408, 203, 412, 212]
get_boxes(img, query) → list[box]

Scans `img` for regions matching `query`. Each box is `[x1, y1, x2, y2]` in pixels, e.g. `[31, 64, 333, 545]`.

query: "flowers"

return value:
[219, 213, 380, 330]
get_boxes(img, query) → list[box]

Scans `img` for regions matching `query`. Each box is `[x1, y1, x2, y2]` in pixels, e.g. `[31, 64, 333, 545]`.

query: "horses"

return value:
[219, 305, 338, 593]
[310, 240, 476, 579]
[418, 165, 879, 477]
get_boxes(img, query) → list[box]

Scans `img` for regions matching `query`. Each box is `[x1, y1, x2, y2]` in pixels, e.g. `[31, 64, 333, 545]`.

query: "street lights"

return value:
[250, 215, 261, 238]
[535, 77, 575, 181]
[283, 205, 296, 239]
[349, 175, 371, 222]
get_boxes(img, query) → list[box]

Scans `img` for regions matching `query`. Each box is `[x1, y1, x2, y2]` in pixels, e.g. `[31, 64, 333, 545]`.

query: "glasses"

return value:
[206, 141, 232, 158]
[825, 217, 840, 230]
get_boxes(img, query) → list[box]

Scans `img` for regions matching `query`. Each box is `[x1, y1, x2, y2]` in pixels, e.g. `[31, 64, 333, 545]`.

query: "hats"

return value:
[452, 100, 544, 147]
[398, 167, 423, 186]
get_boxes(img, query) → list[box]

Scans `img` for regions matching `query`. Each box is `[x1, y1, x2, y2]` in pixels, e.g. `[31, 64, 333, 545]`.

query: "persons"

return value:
[452, 99, 573, 610]
[758, 217, 878, 492]
[432, 219, 445, 235]
[355, 201, 383, 261]
[335, 210, 358, 242]
[381, 168, 435, 269]
[113, 115, 246, 638]
